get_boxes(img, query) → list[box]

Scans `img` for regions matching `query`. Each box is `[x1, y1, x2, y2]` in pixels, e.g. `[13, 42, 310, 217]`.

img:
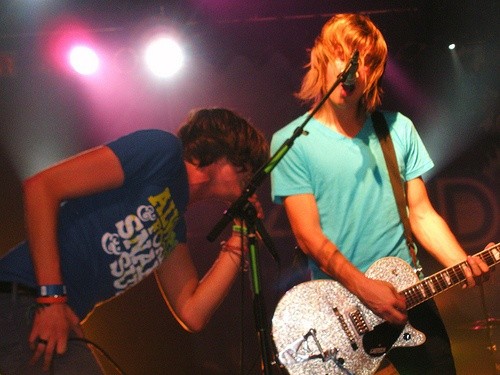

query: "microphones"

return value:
[240, 201, 283, 265]
[342, 51, 360, 91]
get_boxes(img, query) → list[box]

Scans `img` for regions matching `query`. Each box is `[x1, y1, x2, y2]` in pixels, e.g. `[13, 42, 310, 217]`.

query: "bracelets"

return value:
[36, 283, 67, 297]
[232, 225, 248, 233]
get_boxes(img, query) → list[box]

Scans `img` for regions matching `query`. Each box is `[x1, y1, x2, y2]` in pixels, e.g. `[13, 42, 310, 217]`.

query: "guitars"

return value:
[271, 241, 500, 375]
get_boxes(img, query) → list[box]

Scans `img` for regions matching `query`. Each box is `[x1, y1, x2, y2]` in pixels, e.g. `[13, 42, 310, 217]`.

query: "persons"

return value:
[0, 109, 270, 375]
[270, 13, 496, 375]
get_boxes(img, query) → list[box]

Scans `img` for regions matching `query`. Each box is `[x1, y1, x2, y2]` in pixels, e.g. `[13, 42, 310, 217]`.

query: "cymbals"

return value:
[453, 317, 500, 330]
